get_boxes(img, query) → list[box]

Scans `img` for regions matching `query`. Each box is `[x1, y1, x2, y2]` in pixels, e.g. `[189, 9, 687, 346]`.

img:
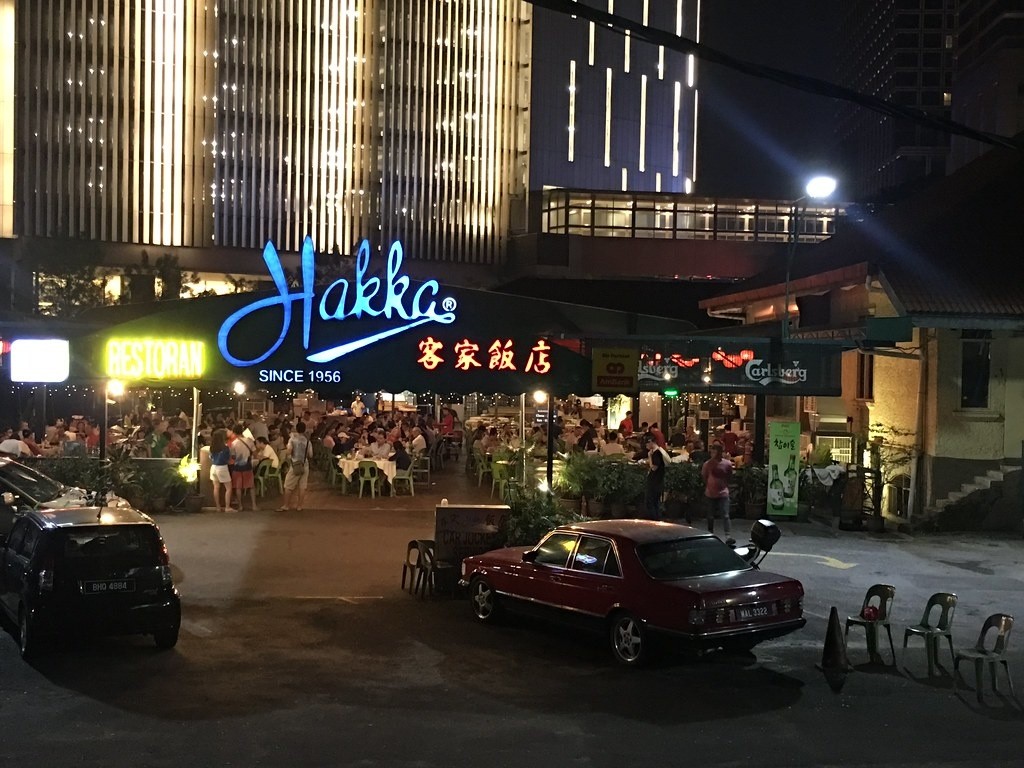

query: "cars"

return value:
[458, 519, 806, 674]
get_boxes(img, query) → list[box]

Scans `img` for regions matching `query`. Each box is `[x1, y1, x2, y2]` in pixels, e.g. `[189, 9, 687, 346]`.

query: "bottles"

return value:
[780, 454, 797, 499]
[351, 447, 355, 458]
[770, 465, 784, 511]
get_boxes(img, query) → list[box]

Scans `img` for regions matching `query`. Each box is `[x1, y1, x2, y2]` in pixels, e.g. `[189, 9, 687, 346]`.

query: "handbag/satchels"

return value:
[292, 460, 305, 475]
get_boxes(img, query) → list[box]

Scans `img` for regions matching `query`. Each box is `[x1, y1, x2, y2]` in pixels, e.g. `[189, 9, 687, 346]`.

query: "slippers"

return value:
[275, 507, 289, 512]
[217, 509, 221, 512]
[296, 509, 303, 512]
[225, 508, 237, 513]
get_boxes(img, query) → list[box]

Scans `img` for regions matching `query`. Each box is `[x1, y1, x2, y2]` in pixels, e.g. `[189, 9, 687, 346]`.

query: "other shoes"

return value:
[726, 538, 736, 544]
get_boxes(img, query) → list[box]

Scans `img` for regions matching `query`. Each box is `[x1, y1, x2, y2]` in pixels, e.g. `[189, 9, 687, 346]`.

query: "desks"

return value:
[336, 459, 397, 495]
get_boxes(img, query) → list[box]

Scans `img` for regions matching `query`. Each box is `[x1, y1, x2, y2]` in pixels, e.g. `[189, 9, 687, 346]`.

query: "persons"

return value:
[319, 395, 437, 498]
[201, 407, 318, 513]
[471, 418, 544, 469]
[701, 445, 733, 538]
[639, 431, 665, 515]
[433, 407, 454, 462]
[120, 407, 192, 457]
[554, 410, 743, 465]
[0, 415, 111, 456]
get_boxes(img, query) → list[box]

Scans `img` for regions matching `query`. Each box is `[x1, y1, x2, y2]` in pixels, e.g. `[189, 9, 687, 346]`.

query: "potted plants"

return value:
[105, 443, 205, 513]
[852, 422, 923, 531]
[554, 446, 834, 521]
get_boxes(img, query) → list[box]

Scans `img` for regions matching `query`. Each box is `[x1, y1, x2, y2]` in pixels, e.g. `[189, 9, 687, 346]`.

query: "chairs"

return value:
[402, 540, 458, 600]
[844, 583, 895, 667]
[902, 592, 959, 678]
[251, 430, 518, 499]
[954, 614, 1016, 702]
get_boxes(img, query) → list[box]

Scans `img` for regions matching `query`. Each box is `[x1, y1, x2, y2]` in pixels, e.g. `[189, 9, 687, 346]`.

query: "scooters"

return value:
[725, 519, 781, 569]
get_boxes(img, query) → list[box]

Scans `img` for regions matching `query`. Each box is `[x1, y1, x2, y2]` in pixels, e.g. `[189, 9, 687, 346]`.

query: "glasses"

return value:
[648, 441, 650, 443]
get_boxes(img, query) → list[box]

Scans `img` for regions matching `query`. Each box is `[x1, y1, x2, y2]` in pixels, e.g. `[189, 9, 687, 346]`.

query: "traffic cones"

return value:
[815, 607, 854, 672]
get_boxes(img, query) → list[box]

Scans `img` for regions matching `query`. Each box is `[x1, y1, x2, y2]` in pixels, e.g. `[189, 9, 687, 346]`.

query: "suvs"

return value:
[0, 457, 132, 542]
[0, 507, 182, 659]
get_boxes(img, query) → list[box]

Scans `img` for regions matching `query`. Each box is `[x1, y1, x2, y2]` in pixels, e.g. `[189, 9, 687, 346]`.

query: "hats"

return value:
[338, 432, 350, 438]
